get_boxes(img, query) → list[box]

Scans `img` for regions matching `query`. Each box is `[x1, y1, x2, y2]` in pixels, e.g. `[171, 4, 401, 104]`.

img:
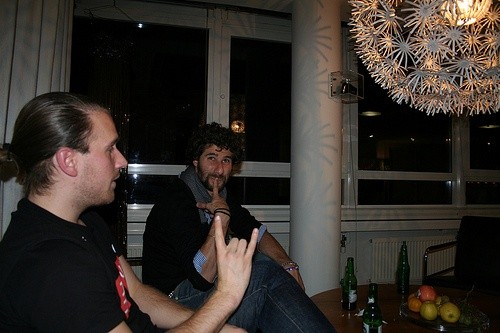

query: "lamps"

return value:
[349, 0, 500, 116]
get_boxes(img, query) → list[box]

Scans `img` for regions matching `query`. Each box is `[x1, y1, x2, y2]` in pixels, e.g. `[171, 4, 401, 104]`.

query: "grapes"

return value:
[458, 311, 480, 326]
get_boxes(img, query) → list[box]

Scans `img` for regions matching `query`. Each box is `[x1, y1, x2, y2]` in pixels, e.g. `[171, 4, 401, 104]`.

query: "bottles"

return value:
[397, 245, 411, 295]
[362, 283, 382, 333]
[342, 257, 357, 311]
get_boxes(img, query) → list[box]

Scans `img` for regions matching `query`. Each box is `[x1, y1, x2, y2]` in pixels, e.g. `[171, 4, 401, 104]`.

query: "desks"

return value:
[309, 284, 500, 333]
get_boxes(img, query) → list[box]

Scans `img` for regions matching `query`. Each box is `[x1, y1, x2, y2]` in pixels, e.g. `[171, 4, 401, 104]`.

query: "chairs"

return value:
[422, 216, 500, 296]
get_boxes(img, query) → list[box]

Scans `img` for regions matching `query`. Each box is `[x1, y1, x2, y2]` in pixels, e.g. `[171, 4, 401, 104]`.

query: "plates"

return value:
[400, 300, 492, 333]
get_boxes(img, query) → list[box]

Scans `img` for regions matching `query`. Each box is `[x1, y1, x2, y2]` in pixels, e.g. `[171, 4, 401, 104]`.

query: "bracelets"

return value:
[280, 261, 297, 268]
[208, 234, 215, 239]
[285, 266, 299, 271]
[214, 208, 231, 218]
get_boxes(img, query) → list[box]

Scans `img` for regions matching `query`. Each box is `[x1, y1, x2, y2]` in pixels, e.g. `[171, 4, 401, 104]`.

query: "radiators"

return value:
[371, 236, 456, 284]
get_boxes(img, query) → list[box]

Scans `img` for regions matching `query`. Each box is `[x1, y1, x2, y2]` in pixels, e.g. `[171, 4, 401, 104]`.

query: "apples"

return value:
[407, 284, 461, 323]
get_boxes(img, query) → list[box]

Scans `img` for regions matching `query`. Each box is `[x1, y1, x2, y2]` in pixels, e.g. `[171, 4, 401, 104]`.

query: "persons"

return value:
[141, 122, 305, 295]
[0, 90, 337, 333]
